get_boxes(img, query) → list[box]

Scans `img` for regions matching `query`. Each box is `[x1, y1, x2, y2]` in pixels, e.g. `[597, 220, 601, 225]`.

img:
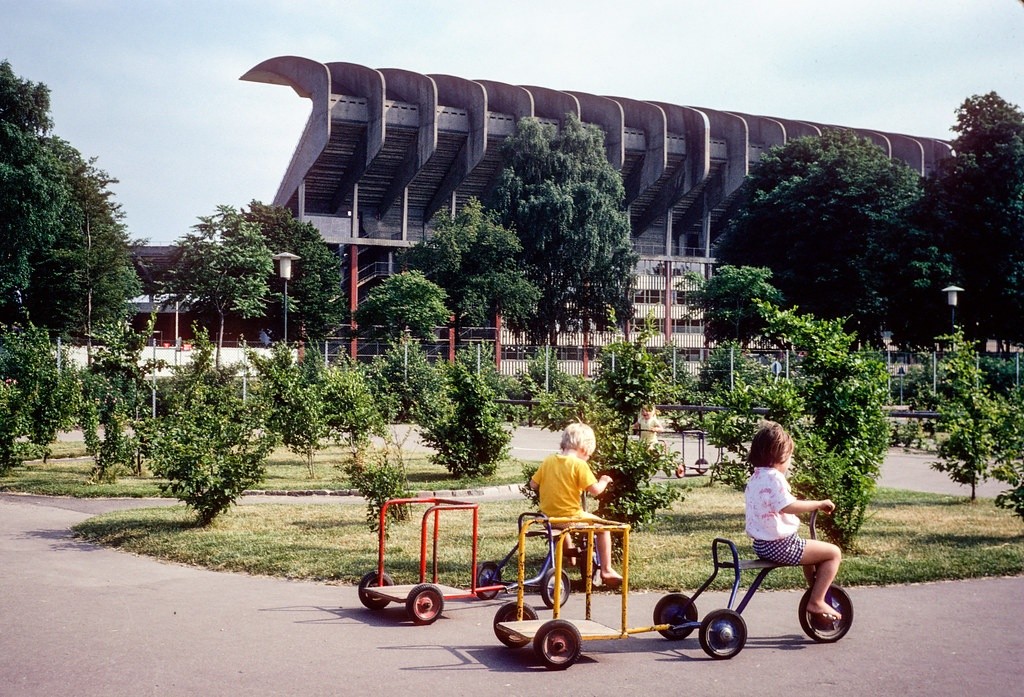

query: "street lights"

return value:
[941, 285, 965, 358]
[274, 252, 302, 354]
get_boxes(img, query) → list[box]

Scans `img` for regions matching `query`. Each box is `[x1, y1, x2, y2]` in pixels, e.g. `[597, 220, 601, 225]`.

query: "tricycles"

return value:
[630, 428, 686, 478]
[652, 505, 855, 662]
[472, 482, 624, 609]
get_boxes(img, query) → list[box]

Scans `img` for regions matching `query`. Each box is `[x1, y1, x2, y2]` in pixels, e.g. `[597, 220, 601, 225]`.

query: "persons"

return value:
[746, 423, 842, 620]
[530, 423, 623, 587]
